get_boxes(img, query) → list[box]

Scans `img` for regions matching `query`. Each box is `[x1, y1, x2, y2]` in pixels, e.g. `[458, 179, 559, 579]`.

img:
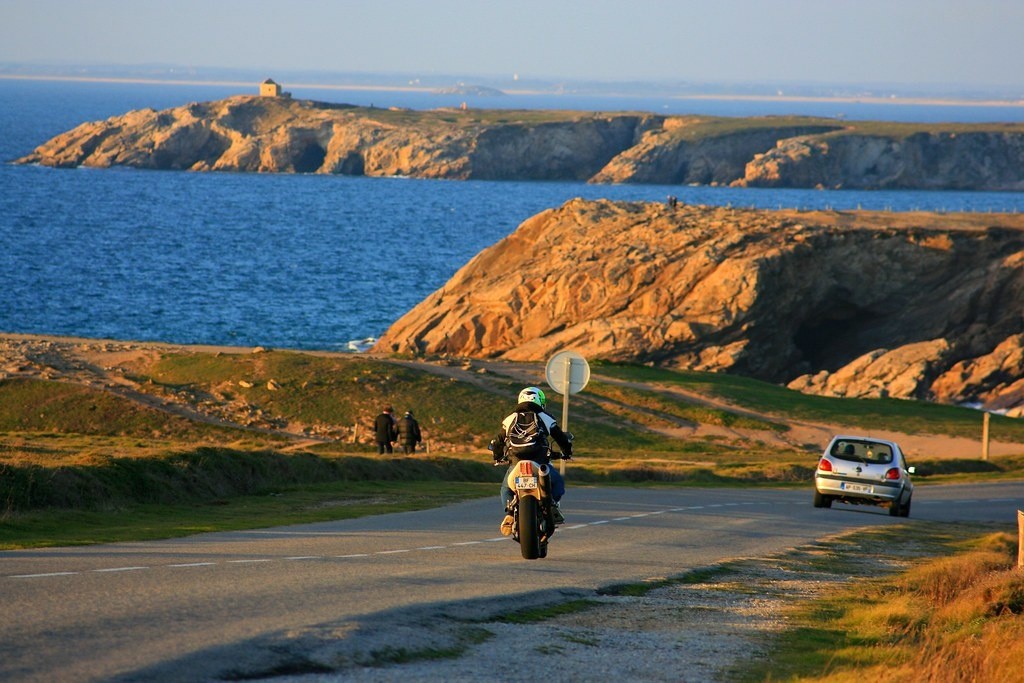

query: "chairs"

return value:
[836, 444, 862, 463]
[873, 452, 890, 463]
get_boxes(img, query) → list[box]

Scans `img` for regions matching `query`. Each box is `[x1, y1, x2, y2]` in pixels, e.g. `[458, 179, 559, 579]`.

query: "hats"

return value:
[405, 410, 413, 417]
[383, 405, 393, 412]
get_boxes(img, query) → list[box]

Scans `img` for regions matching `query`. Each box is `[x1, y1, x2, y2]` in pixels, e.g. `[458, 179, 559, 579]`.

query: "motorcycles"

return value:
[487, 431, 575, 559]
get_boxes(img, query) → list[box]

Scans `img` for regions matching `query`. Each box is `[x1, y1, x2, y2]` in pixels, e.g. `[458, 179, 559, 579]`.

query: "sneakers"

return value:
[501, 514, 514, 536]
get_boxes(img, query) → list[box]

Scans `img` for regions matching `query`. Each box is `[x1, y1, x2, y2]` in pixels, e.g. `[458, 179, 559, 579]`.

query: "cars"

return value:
[814, 435, 916, 519]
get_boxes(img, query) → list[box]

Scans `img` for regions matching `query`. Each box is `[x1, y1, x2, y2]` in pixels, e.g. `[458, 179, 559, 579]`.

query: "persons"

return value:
[373, 405, 399, 455]
[842, 444, 855, 455]
[403, 411, 422, 455]
[490, 386, 573, 536]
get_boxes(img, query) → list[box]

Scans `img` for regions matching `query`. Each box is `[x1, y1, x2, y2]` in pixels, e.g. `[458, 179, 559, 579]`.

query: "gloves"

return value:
[418, 441, 422, 447]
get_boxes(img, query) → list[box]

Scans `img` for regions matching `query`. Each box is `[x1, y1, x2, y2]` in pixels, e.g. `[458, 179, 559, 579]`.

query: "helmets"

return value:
[518, 387, 546, 409]
